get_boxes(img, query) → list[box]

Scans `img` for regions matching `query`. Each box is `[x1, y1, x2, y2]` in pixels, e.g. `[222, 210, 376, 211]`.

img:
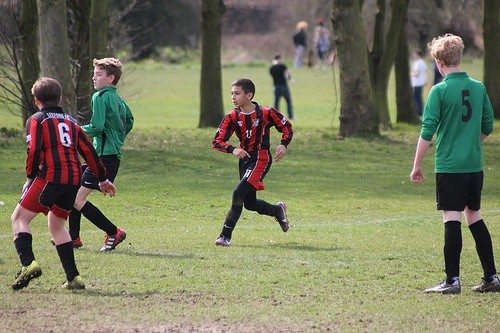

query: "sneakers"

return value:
[471, 275, 500, 293]
[215, 234, 231, 245]
[99, 227, 126, 253]
[60, 275, 85, 289]
[50, 236, 83, 249]
[425, 280, 461, 295]
[12, 260, 42, 291]
[274, 202, 289, 232]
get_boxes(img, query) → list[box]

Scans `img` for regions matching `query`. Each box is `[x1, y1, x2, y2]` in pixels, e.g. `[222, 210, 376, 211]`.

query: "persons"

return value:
[409, 34, 500, 294]
[212, 78, 293, 246]
[270, 19, 443, 122]
[50, 57, 134, 253]
[12, 77, 116, 289]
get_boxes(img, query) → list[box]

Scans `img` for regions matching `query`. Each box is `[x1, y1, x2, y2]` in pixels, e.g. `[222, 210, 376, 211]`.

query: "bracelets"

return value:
[99, 181, 110, 187]
